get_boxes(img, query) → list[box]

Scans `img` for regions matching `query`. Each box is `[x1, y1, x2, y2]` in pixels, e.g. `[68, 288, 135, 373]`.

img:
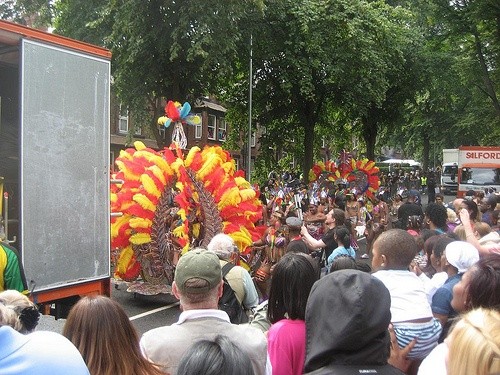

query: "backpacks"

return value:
[221, 263, 248, 325]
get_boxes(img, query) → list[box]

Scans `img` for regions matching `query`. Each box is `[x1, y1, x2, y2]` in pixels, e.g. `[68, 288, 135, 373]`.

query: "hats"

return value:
[446, 241, 479, 273]
[286, 217, 303, 229]
[175, 248, 222, 298]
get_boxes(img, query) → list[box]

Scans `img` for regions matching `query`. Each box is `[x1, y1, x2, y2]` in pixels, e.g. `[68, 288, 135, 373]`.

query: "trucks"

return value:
[441, 149, 459, 195]
[456, 146, 500, 199]
[0, 19, 123, 320]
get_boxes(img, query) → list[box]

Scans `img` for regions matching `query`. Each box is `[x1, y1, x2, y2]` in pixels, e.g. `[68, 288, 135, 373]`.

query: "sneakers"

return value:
[360, 254, 369, 258]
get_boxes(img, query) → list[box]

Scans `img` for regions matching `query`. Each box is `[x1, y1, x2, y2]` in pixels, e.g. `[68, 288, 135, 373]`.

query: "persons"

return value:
[370, 229, 441, 360]
[0, 241, 29, 297]
[63, 295, 169, 375]
[207, 234, 259, 324]
[178, 254, 322, 375]
[0, 290, 90, 375]
[302, 269, 417, 375]
[138, 248, 267, 375]
[260, 164, 443, 283]
[388, 188, 500, 375]
[252, 210, 290, 304]
